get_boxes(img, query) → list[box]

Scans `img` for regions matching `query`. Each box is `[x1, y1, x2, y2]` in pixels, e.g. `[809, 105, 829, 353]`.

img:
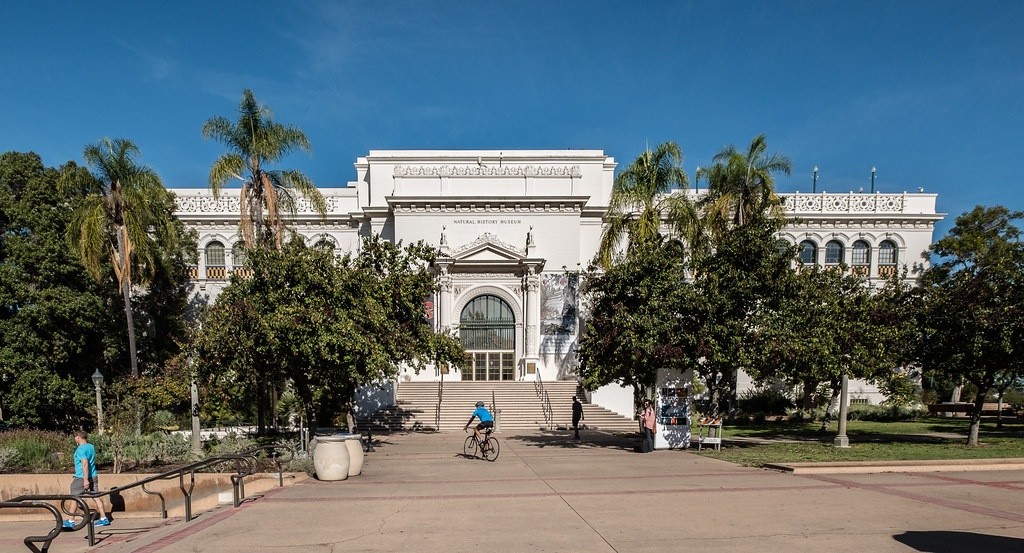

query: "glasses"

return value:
[74, 434, 78, 437]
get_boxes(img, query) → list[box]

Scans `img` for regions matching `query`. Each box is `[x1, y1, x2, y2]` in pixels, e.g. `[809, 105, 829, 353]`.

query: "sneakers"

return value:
[62, 519, 75, 528]
[94, 517, 111, 526]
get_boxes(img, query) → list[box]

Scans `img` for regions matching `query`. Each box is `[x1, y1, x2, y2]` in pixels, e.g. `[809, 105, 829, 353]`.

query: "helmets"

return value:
[475, 401, 484, 406]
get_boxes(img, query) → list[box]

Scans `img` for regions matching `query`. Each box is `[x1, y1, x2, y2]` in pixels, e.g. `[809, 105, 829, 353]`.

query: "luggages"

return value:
[633, 415, 648, 453]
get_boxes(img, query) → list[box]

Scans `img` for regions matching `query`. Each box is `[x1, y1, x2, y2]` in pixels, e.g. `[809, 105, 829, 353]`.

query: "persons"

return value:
[636, 398, 657, 452]
[464, 401, 495, 459]
[569, 395, 586, 444]
[60, 430, 112, 528]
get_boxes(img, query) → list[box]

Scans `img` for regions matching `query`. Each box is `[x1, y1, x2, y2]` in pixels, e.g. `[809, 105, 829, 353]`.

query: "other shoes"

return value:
[482, 453, 488, 460]
[478, 439, 483, 446]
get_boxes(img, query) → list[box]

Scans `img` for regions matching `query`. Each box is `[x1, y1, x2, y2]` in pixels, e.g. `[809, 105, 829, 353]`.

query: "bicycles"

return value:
[465, 426, 510, 462]
